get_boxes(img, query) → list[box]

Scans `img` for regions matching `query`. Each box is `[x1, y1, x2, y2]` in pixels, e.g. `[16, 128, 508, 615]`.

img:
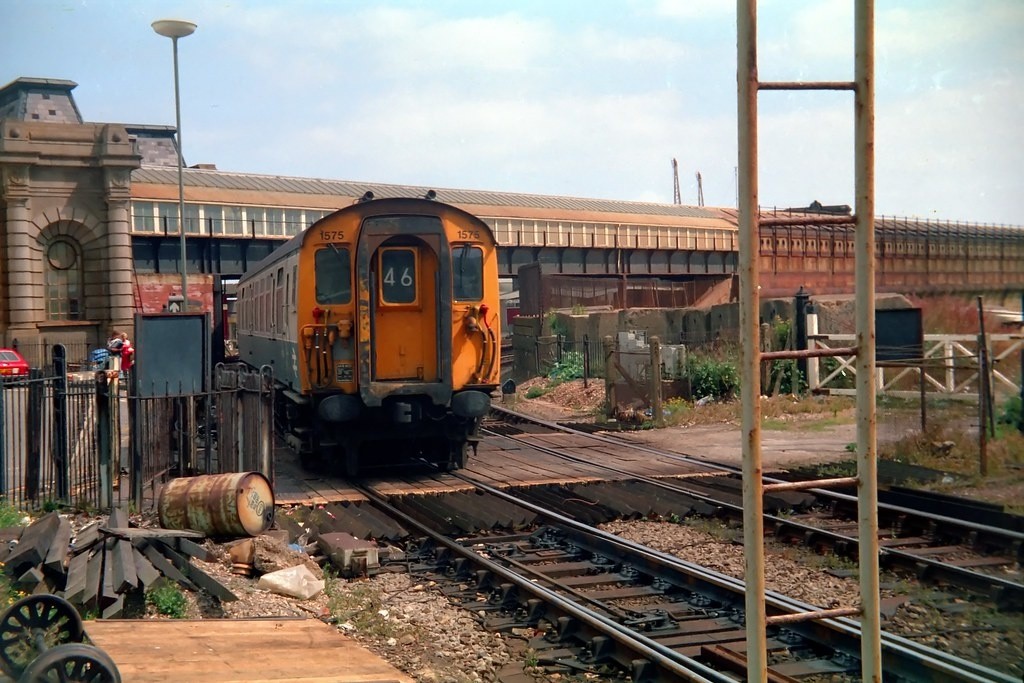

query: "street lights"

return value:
[152, 17, 198, 312]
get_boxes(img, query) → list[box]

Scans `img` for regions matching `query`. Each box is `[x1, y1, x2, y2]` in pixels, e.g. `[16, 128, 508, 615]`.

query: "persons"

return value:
[120, 333, 135, 379]
[105, 331, 123, 357]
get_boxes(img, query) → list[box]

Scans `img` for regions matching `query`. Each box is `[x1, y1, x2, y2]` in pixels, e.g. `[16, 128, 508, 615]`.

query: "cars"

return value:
[0, 349, 30, 387]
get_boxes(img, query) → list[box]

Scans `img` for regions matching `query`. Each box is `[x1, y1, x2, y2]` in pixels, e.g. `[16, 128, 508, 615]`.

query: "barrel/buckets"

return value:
[158, 472, 275, 537]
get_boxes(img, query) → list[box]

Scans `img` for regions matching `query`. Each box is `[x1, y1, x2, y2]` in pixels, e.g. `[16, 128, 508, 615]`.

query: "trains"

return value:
[234, 188, 517, 477]
